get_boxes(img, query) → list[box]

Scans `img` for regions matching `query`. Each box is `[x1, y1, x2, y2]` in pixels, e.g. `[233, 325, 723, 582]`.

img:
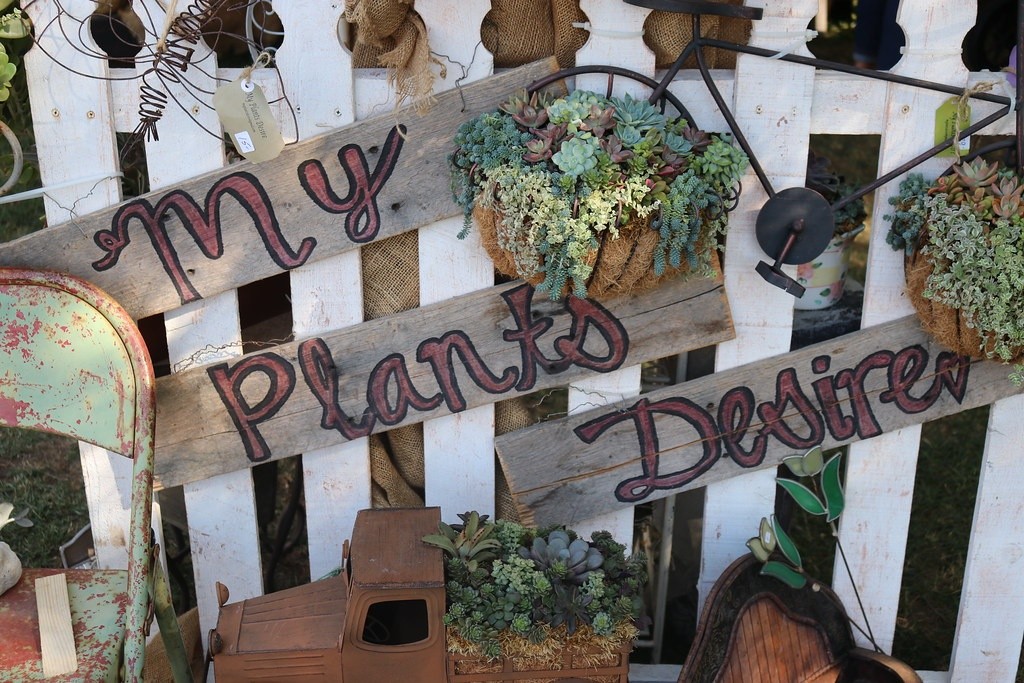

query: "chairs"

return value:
[0, 267, 192, 683]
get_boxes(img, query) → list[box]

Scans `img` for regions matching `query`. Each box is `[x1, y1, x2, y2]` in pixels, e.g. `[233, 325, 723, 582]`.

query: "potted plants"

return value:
[448, 86, 750, 300]
[794, 147, 867, 311]
[882, 154, 1024, 386]
[419, 508, 650, 683]
